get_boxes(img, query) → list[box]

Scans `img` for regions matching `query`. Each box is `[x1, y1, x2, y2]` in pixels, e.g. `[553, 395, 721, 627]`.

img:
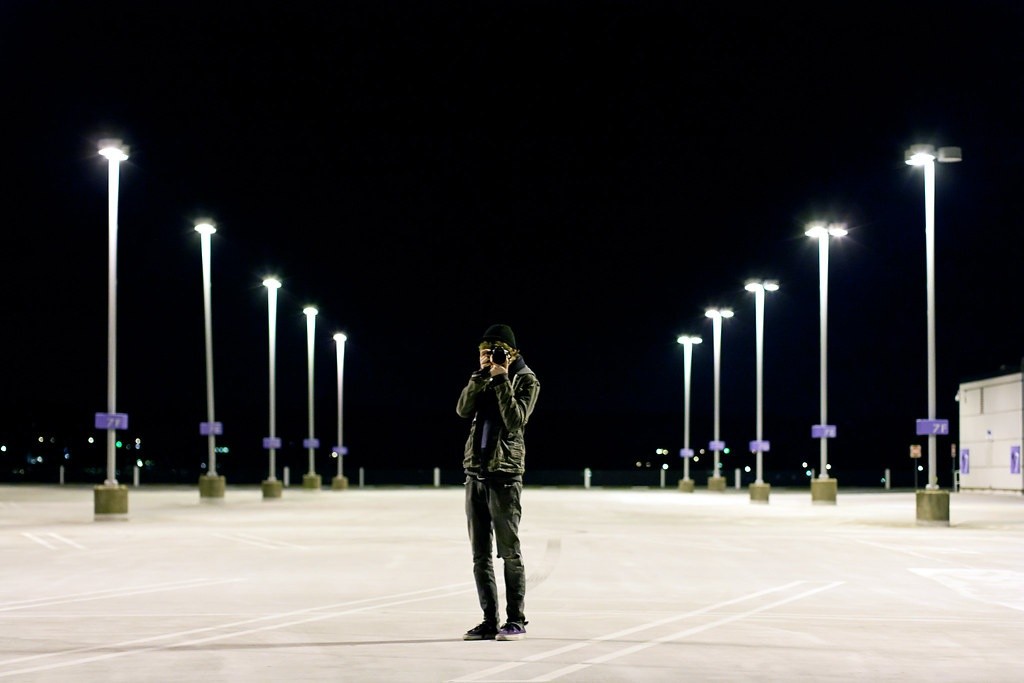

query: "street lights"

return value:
[262, 276, 283, 481]
[97, 137, 130, 487]
[677, 335, 704, 481]
[903, 142, 962, 489]
[302, 307, 319, 476]
[744, 278, 780, 485]
[805, 217, 848, 480]
[705, 306, 735, 479]
[333, 334, 348, 478]
[193, 216, 218, 478]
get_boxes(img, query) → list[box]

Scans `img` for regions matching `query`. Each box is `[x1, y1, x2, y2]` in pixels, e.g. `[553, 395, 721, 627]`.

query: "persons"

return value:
[456, 324, 540, 641]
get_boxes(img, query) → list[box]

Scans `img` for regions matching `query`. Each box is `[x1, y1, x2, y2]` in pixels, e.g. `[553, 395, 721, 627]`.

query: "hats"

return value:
[483, 324, 516, 349]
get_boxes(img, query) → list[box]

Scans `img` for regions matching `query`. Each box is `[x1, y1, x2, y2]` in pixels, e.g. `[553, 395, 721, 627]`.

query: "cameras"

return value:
[486, 344, 509, 365]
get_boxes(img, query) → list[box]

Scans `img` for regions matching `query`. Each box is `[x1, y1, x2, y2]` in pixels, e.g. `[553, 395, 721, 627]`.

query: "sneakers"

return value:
[495, 624, 526, 640]
[463, 621, 501, 640]
